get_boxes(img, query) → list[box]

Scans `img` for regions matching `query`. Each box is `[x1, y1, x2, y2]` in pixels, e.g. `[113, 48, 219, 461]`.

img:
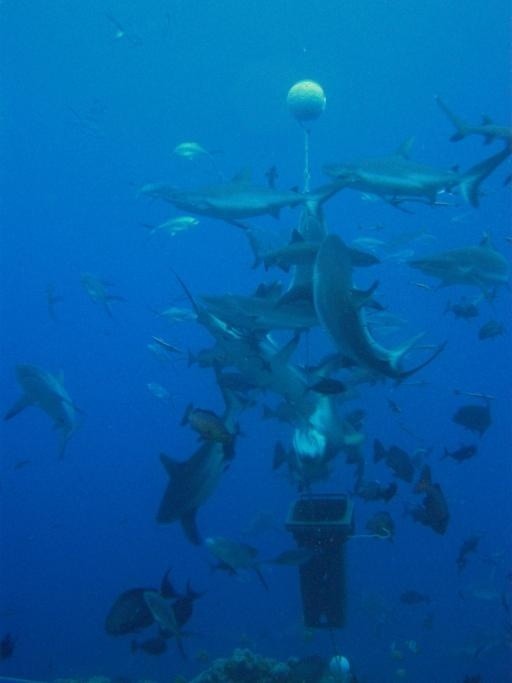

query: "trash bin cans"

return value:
[285, 493, 355, 629]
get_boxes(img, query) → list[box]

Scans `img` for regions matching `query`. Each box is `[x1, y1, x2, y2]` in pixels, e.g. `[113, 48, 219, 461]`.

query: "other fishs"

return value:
[141, 95, 512, 590]
